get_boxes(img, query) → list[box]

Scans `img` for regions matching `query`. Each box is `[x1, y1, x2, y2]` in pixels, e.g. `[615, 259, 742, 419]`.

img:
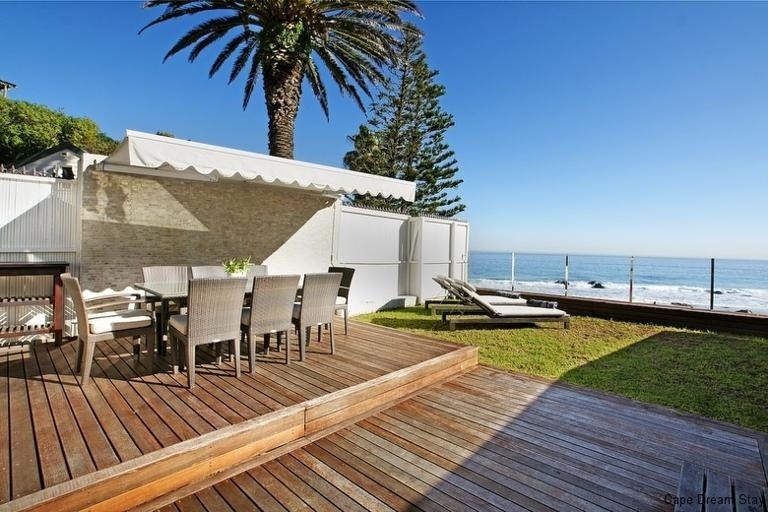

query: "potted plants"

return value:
[222, 255, 251, 278]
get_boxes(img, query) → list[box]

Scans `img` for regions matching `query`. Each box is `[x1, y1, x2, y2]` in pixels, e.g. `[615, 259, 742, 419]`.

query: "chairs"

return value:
[229, 275, 301, 373]
[277, 271, 343, 361]
[425, 273, 571, 331]
[60, 272, 155, 385]
[168, 278, 246, 389]
[190, 265, 227, 280]
[243, 265, 268, 278]
[141, 265, 188, 352]
[326, 267, 355, 336]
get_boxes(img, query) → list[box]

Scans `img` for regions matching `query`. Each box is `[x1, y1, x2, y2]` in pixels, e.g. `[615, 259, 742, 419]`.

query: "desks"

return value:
[133, 281, 323, 371]
